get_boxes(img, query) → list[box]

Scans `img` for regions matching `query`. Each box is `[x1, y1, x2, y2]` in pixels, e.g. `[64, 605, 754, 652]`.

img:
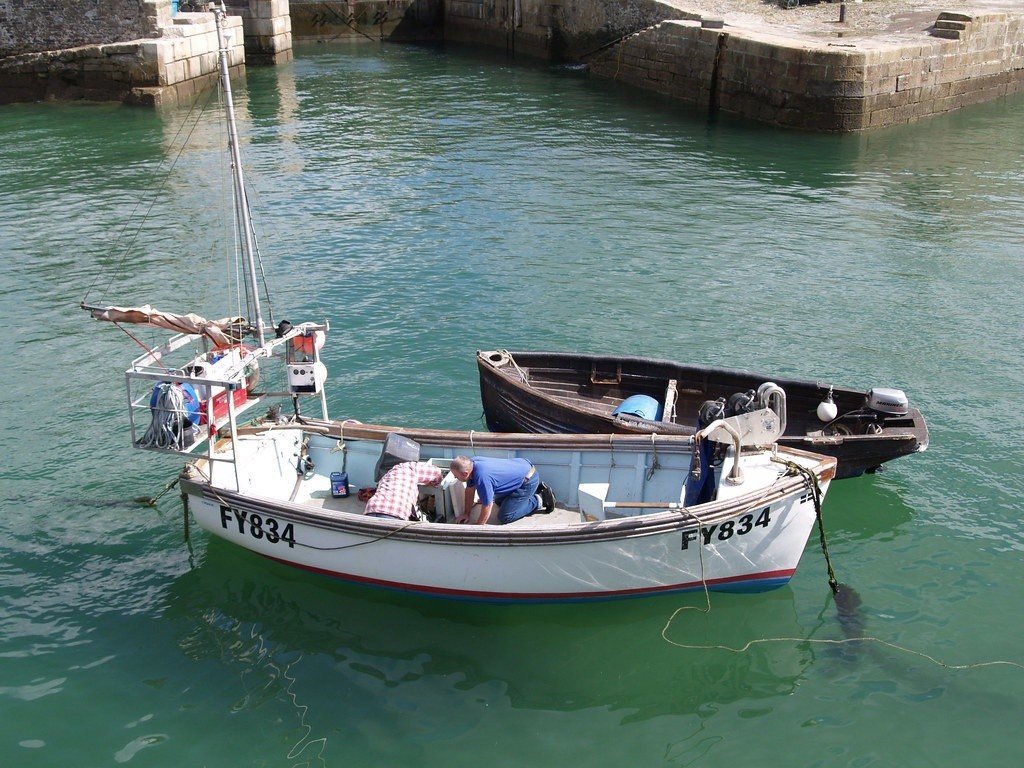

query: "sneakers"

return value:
[542, 487, 554, 514]
[537, 481, 557, 503]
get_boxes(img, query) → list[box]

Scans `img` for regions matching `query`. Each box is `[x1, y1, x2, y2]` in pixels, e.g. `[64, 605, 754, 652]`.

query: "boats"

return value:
[476, 350, 930, 481]
[79, 1, 836, 603]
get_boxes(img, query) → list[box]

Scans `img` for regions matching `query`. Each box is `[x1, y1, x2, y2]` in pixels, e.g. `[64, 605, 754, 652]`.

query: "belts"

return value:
[524, 465, 536, 482]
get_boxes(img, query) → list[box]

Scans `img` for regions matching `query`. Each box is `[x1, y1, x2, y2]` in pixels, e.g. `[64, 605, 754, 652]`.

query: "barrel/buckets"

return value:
[330, 472, 349, 498]
[610, 395, 663, 421]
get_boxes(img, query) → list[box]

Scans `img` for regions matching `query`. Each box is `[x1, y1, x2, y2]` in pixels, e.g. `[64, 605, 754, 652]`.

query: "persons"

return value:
[450, 455, 556, 525]
[363, 460, 443, 522]
